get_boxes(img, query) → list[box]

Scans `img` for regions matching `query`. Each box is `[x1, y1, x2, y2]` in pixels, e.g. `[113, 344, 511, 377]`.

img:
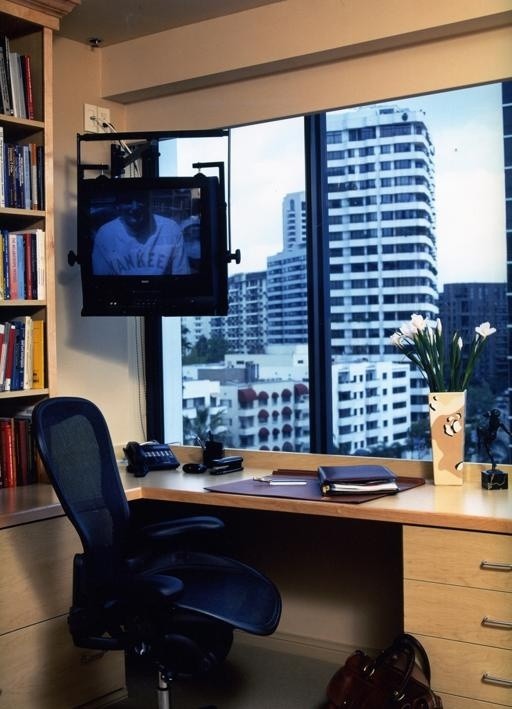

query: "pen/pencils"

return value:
[197, 431, 213, 452]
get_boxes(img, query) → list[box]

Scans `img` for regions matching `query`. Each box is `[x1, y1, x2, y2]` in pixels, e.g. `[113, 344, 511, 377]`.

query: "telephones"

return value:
[123, 442, 180, 470]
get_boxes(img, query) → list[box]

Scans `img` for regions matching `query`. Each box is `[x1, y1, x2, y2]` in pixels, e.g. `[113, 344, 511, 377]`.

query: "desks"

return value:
[0, 457, 511, 709]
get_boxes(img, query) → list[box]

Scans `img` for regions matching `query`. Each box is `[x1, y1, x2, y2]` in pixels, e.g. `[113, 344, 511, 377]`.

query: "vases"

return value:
[428, 390, 466, 486]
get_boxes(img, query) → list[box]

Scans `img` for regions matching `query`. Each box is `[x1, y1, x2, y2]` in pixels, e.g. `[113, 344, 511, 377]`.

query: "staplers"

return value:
[211, 456, 243, 475]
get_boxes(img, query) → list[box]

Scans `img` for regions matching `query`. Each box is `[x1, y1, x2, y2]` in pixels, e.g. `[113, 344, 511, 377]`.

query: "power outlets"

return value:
[83, 102, 111, 135]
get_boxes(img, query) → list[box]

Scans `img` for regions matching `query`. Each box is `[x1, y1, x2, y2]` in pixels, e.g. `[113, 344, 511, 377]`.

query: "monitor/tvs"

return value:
[76, 175, 231, 317]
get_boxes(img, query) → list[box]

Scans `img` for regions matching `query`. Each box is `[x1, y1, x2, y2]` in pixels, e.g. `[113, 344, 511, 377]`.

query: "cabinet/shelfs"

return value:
[0, 0, 56, 485]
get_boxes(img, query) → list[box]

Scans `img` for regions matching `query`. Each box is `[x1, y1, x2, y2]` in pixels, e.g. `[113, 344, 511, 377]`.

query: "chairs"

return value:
[32, 396, 282, 709]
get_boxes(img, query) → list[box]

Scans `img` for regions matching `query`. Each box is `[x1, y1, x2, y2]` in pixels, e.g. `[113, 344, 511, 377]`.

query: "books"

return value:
[317, 463, 400, 496]
[0, 35, 48, 489]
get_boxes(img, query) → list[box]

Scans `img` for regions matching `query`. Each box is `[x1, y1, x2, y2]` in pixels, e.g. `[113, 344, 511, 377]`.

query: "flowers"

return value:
[390, 313, 496, 392]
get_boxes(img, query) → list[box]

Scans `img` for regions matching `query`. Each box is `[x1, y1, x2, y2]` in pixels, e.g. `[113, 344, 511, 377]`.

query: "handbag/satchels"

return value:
[326, 634, 444, 708]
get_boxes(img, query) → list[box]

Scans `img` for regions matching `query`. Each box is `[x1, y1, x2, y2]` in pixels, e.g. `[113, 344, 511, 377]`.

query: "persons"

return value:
[90, 188, 191, 277]
